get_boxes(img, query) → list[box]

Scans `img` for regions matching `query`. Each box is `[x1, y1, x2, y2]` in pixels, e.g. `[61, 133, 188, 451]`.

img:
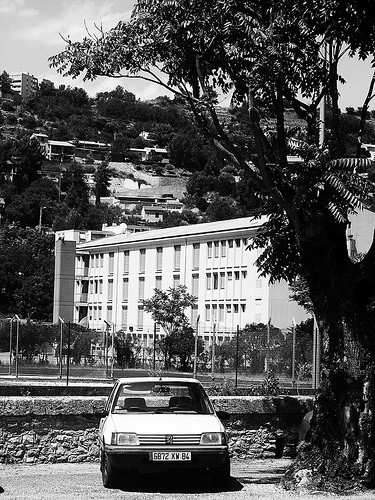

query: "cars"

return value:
[99, 376, 230, 490]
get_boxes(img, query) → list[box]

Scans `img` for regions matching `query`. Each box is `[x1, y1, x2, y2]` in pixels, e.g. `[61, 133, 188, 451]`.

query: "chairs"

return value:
[123, 398, 147, 410]
[169, 396, 192, 410]
